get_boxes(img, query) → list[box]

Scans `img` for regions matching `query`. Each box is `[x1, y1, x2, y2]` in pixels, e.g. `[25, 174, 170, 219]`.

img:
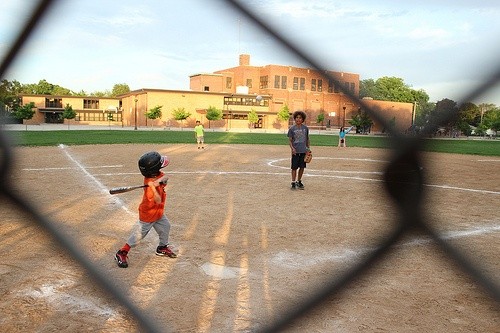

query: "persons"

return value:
[194, 121, 206, 150]
[288, 111, 311, 191]
[114, 152, 178, 268]
[338, 126, 353, 150]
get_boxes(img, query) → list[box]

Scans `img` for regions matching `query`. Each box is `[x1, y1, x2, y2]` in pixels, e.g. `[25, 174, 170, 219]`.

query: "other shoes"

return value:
[290, 182, 296, 191]
[296, 181, 305, 190]
[202, 147, 204, 149]
[198, 147, 200, 149]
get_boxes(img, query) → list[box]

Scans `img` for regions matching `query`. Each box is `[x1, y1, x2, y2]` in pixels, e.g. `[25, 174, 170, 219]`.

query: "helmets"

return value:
[138, 151, 169, 177]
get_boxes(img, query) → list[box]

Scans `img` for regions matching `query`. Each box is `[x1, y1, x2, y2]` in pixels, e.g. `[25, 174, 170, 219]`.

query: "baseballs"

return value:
[256, 95, 262, 101]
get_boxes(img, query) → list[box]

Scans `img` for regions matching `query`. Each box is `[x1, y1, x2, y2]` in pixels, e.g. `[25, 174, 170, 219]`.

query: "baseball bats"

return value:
[109, 180, 167, 195]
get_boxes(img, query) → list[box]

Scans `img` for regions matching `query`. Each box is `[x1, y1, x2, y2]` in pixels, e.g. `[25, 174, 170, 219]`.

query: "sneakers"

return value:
[114, 250, 129, 268]
[155, 245, 177, 258]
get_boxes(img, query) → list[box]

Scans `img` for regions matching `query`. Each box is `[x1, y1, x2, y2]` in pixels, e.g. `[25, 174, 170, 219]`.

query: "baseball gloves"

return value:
[341, 139, 344, 143]
[304, 151, 313, 163]
